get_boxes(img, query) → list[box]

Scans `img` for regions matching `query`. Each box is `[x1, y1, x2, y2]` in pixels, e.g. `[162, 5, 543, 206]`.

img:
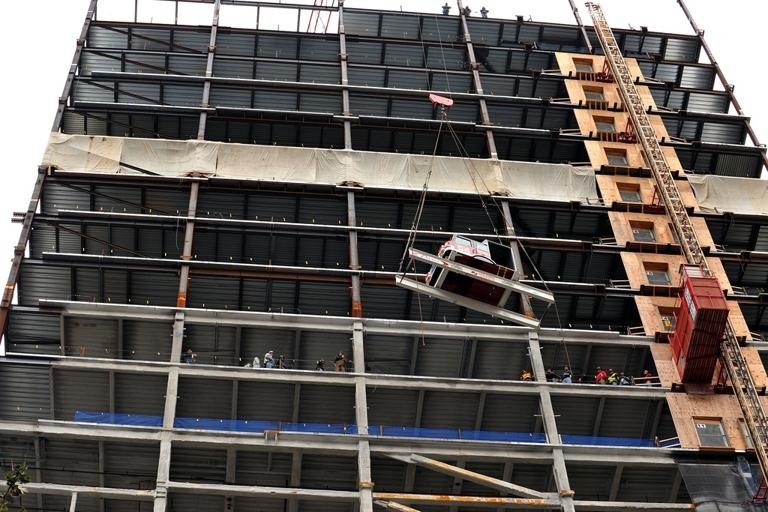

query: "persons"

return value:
[442, 3, 488, 19]
[184, 347, 198, 364]
[244, 348, 347, 371]
[520, 366, 655, 387]
